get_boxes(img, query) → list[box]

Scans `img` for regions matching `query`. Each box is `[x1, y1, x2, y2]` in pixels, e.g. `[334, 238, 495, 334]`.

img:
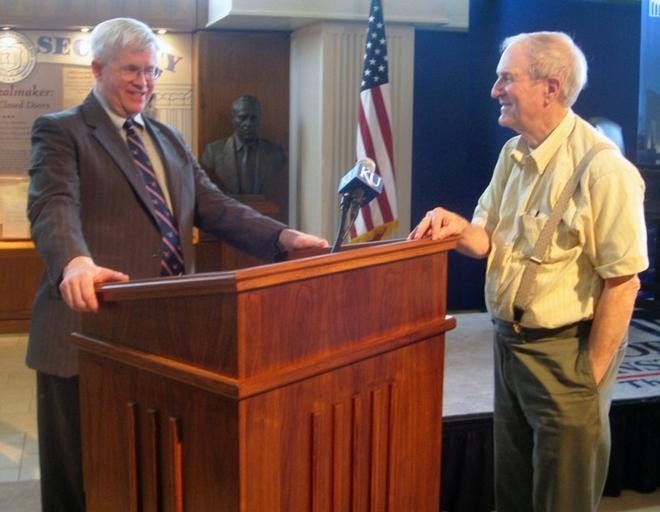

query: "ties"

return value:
[123, 119, 185, 277]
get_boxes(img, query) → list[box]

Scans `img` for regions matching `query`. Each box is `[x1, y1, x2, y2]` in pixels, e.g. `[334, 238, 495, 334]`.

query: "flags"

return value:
[344, 1, 399, 247]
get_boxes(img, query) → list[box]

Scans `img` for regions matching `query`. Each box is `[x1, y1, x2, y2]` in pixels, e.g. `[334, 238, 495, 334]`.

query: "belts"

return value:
[494, 322, 568, 338]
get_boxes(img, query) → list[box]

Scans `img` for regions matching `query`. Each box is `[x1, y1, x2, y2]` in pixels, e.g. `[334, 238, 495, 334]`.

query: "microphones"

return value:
[330, 158, 375, 251]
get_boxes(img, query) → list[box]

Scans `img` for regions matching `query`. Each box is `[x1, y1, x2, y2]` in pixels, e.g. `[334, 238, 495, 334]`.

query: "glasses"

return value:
[116, 65, 163, 80]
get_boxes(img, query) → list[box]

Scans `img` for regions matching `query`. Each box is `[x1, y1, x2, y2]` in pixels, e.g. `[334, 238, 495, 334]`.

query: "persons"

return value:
[406, 28, 653, 512]
[198, 94, 288, 268]
[25, 16, 332, 512]
